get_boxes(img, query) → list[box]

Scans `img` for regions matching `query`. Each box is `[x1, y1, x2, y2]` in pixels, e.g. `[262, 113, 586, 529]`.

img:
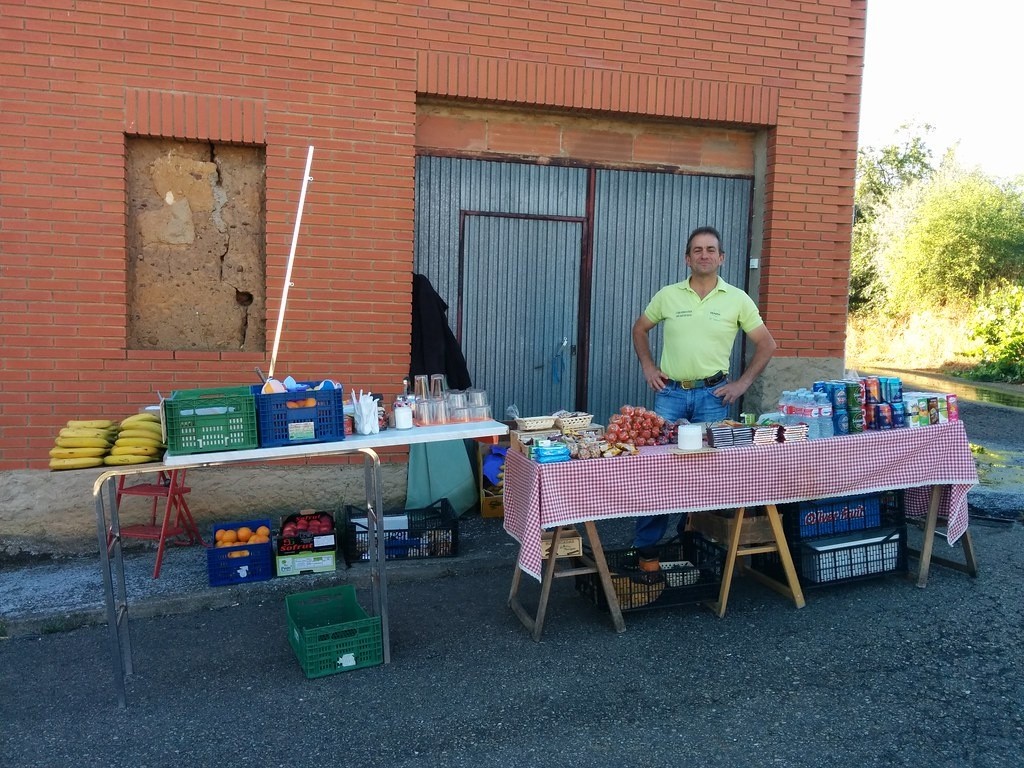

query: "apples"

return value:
[280, 516, 332, 537]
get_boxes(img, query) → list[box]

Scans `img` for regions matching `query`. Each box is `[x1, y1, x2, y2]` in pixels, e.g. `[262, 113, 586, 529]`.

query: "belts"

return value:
[661, 374, 727, 390]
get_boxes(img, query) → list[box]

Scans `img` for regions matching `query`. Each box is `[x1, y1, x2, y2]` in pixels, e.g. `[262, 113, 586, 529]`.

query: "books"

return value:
[706, 422, 810, 449]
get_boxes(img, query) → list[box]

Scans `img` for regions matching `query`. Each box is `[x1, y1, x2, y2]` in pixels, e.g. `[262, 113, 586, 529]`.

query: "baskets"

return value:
[750, 489, 909, 588]
[277, 513, 337, 556]
[571, 531, 735, 613]
[250, 380, 346, 448]
[554, 414, 593, 430]
[285, 586, 384, 678]
[344, 497, 459, 563]
[164, 384, 258, 455]
[206, 517, 275, 587]
[514, 415, 559, 431]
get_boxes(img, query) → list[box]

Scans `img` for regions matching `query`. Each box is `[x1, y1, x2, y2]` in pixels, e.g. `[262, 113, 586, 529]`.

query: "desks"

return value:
[47, 419, 510, 711]
[504, 418, 976, 643]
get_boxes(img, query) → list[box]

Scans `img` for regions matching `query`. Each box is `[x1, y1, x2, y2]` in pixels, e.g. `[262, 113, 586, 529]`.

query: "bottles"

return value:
[779, 388, 834, 439]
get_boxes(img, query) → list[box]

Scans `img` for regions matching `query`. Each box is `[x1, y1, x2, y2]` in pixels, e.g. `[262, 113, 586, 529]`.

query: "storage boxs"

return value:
[251, 380, 345, 448]
[284, 583, 384, 679]
[207, 518, 272, 588]
[770, 523, 908, 590]
[477, 442, 510, 519]
[344, 498, 459, 564]
[276, 512, 336, 577]
[164, 386, 258, 455]
[574, 530, 726, 617]
[754, 487, 906, 541]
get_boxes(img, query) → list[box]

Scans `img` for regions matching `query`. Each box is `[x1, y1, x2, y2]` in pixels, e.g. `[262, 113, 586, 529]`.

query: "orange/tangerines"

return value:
[215, 525, 270, 557]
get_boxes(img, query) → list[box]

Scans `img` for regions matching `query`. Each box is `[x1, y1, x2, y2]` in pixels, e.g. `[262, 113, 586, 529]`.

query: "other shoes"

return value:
[638, 569, 663, 585]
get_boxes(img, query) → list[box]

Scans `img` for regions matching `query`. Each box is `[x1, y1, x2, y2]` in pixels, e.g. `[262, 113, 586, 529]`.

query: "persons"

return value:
[620, 227, 776, 569]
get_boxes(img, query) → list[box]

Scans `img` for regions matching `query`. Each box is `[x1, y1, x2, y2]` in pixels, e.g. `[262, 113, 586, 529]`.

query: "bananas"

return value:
[48, 412, 168, 470]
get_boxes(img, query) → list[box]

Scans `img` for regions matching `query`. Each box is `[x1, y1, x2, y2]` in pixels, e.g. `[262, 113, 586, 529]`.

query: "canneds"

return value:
[813, 375, 906, 436]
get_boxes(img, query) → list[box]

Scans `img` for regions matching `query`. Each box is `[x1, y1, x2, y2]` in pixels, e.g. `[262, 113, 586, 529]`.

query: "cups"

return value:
[678, 425, 703, 450]
[414, 374, 492, 428]
